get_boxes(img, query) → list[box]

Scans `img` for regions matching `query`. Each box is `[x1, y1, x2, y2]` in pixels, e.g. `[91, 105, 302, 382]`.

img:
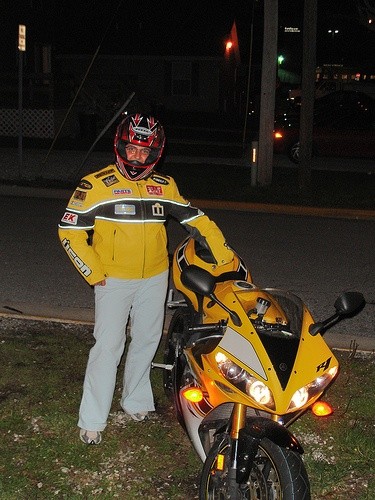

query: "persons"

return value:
[57, 109, 238, 446]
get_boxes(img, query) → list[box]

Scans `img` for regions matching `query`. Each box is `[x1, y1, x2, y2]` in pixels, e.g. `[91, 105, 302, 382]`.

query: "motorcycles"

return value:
[160, 232, 369, 500]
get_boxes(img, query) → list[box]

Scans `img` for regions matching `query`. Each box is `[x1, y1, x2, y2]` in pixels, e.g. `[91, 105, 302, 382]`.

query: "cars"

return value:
[313, 90, 374, 113]
[271, 106, 375, 162]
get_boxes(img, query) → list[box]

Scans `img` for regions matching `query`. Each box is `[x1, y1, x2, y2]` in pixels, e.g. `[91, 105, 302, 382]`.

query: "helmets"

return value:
[114, 112, 166, 181]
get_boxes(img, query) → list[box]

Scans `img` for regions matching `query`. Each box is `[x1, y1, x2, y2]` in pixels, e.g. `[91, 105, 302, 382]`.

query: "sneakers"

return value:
[79, 428, 102, 445]
[131, 413, 145, 421]
